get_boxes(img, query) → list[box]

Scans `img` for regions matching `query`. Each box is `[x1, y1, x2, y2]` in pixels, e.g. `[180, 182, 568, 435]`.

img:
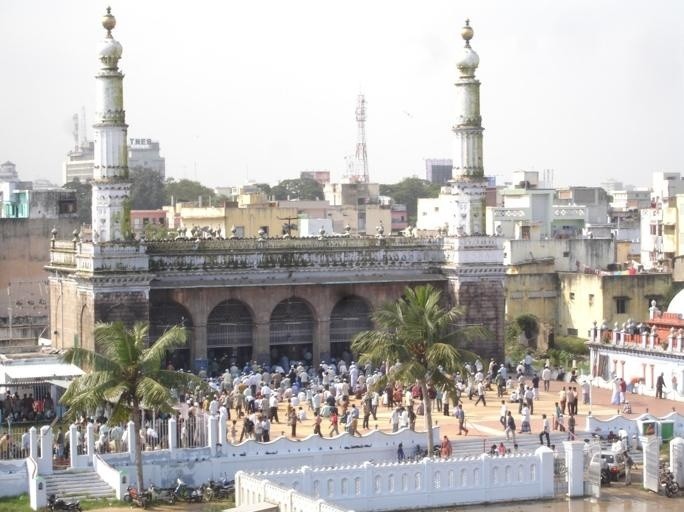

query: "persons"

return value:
[621, 451, 638, 486]
[0, 341, 677, 463]
[599, 454, 611, 487]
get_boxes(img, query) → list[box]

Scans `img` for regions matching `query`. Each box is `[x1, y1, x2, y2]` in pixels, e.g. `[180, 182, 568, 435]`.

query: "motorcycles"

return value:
[659, 469, 679, 497]
[44, 472, 236, 512]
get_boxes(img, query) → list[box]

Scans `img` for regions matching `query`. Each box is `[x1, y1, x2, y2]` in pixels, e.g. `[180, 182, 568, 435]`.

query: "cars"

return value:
[586, 451, 628, 483]
